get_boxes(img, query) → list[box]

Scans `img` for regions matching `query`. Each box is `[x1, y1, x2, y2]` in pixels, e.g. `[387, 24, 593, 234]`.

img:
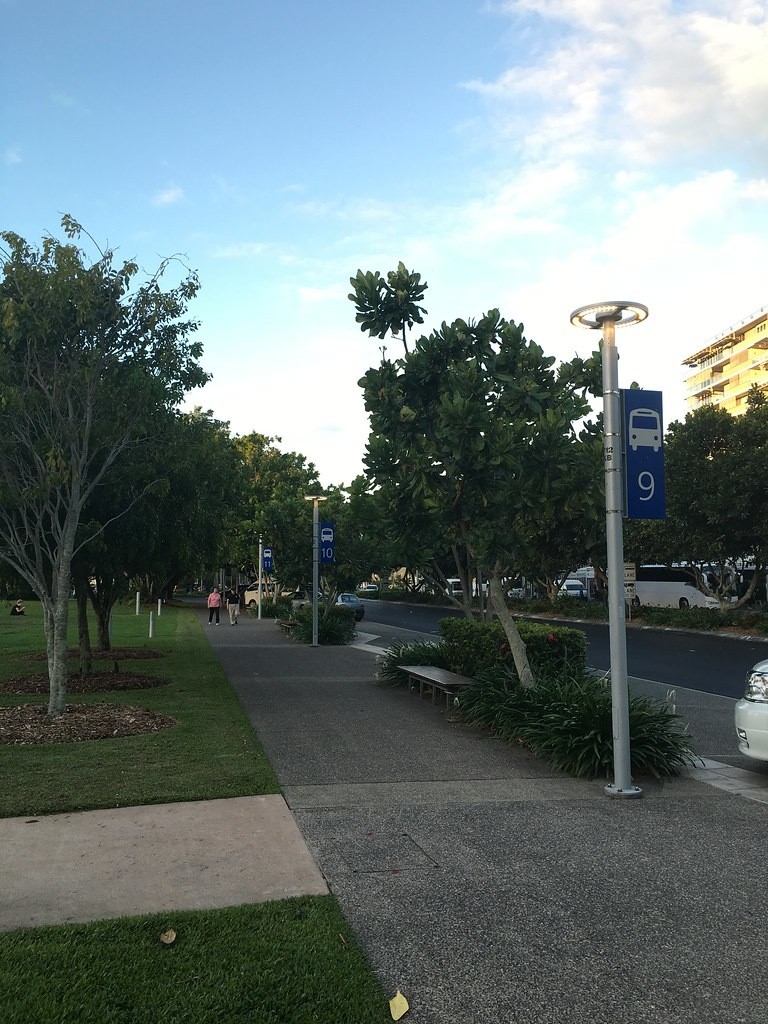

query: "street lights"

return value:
[304, 495, 328, 647]
[569, 302, 649, 799]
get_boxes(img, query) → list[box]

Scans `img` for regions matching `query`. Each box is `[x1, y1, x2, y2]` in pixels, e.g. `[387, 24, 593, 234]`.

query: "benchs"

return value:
[398, 665, 481, 709]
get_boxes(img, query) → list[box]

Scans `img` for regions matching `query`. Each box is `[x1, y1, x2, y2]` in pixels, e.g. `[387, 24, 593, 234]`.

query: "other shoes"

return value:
[215, 622, 219, 626]
[208, 621, 212, 625]
[235, 621, 237, 624]
[230, 624, 234, 626]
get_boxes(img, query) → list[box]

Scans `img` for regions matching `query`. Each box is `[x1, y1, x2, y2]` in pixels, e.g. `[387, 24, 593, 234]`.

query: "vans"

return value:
[553, 580, 587, 599]
[486, 577, 522, 598]
[443, 579, 463, 596]
[243, 576, 299, 608]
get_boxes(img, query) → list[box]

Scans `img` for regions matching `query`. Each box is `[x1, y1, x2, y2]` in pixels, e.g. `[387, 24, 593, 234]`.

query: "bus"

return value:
[558, 567, 600, 599]
[625, 563, 743, 611]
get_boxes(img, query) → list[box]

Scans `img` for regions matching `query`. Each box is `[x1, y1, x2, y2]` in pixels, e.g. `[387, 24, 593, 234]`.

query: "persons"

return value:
[226, 588, 240, 626]
[10, 599, 25, 616]
[207, 587, 222, 625]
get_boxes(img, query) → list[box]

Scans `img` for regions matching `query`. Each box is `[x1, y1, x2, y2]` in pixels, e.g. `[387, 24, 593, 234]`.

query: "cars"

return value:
[735, 659, 768, 761]
[303, 592, 365, 621]
[219, 584, 249, 602]
[287, 592, 322, 610]
[364, 585, 378, 591]
